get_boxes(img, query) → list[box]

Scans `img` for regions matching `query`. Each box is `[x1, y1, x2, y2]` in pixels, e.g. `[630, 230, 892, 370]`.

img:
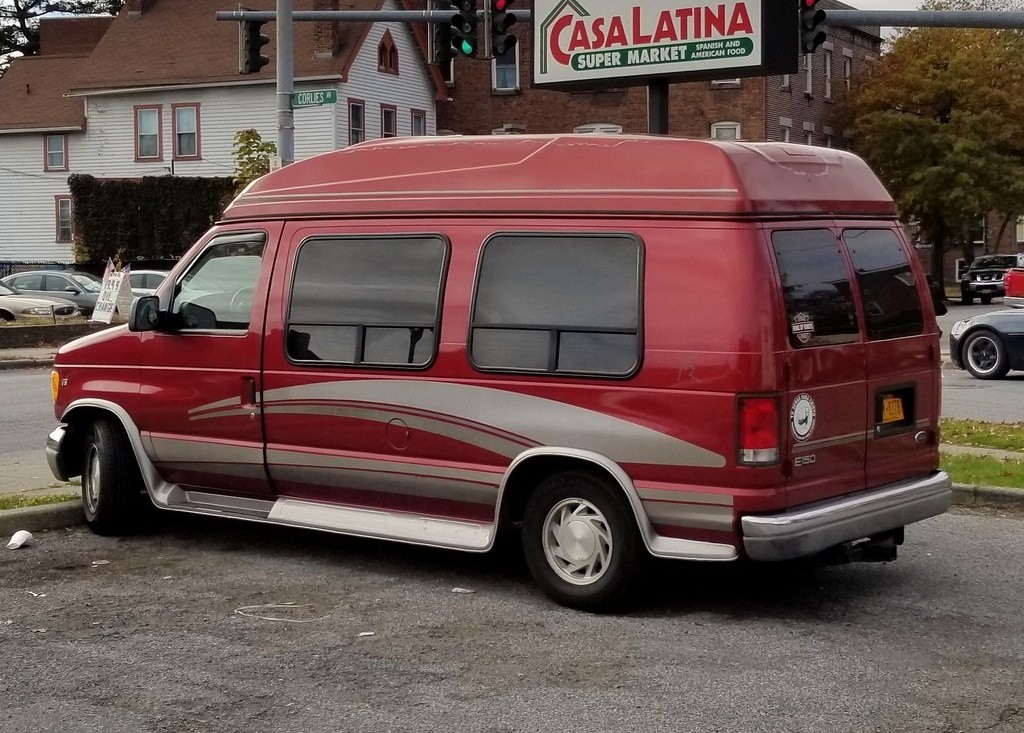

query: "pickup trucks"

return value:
[1002, 267, 1024, 309]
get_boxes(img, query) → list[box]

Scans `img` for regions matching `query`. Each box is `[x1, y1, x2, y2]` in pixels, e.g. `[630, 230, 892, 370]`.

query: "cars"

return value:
[126, 269, 234, 316]
[0, 270, 104, 314]
[948, 307, 1024, 379]
[0, 279, 83, 324]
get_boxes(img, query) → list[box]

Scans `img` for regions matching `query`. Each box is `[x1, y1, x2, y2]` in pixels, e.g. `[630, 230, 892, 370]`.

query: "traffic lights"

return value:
[450, 0, 478, 60]
[483, 1, 517, 60]
[236, 6, 271, 75]
[799, 0, 827, 55]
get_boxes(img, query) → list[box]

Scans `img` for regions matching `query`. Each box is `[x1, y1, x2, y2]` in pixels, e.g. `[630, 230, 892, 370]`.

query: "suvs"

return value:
[959, 253, 1024, 306]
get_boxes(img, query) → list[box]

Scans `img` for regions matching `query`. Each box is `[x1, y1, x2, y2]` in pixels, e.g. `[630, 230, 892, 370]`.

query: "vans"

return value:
[43, 131, 957, 609]
